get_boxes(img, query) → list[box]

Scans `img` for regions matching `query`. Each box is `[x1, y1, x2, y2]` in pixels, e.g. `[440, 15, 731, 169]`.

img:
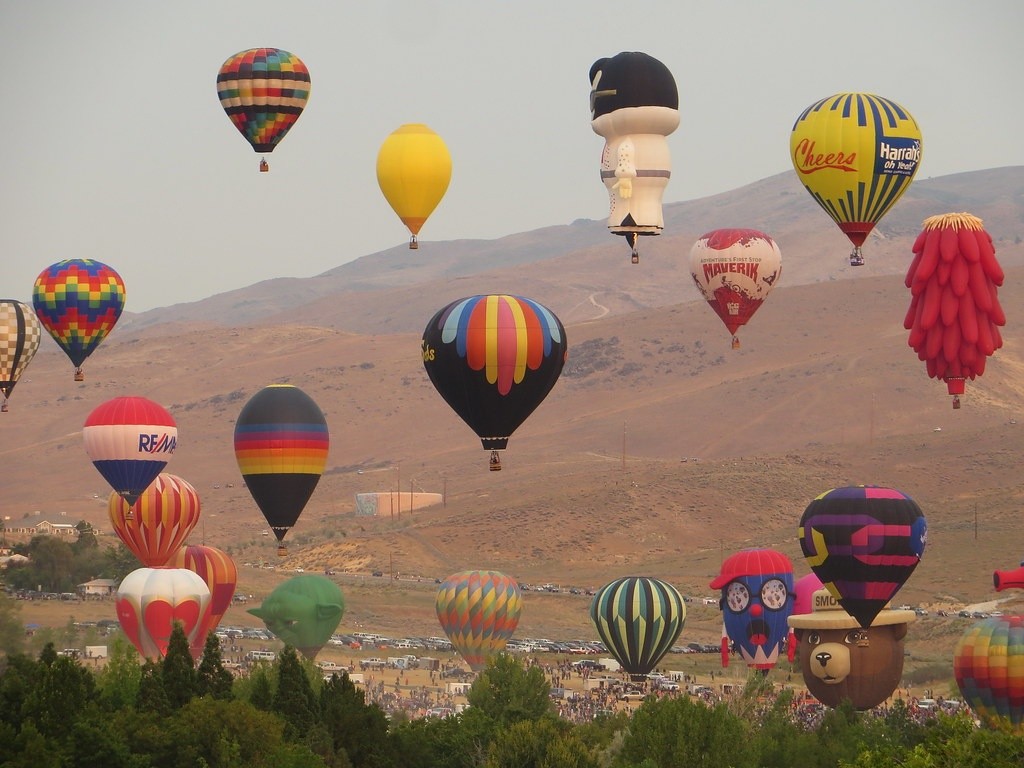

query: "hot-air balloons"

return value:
[0, 258, 126, 413]
[83, 384, 345, 668]
[904, 213, 1006, 411]
[216, 48, 311, 172]
[953, 562, 1024, 739]
[588, 52, 680, 264]
[709, 484, 927, 725]
[789, 93, 923, 266]
[590, 576, 686, 682]
[688, 228, 783, 350]
[420, 294, 567, 469]
[376, 123, 452, 249]
[435, 570, 522, 671]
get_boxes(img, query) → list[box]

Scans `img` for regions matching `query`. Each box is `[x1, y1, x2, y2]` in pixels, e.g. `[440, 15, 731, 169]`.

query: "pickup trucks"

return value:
[622, 691, 647, 702]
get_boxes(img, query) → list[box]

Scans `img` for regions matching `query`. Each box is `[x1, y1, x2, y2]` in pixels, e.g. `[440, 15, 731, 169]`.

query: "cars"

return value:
[683, 596, 694, 602]
[517, 582, 595, 595]
[435, 578, 443, 583]
[658, 680, 680, 692]
[0, 582, 77, 601]
[426, 705, 471, 723]
[704, 597, 717, 605]
[944, 700, 960, 713]
[373, 571, 383, 577]
[918, 699, 939, 712]
[321, 569, 335, 575]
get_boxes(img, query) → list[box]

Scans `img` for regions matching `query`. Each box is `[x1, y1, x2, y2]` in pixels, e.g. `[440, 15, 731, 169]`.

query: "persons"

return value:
[71, 648, 97, 666]
[872, 689, 977, 724]
[512, 653, 832, 733]
[348, 657, 479, 720]
[219, 634, 266, 677]
[493, 457, 497, 463]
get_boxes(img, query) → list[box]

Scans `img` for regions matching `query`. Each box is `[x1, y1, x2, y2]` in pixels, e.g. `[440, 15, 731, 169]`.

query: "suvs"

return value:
[899, 605, 1003, 619]
[57, 649, 80, 657]
[211, 624, 733, 682]
[250, 562, 274, 571]
[294, 567, 304, 573]
